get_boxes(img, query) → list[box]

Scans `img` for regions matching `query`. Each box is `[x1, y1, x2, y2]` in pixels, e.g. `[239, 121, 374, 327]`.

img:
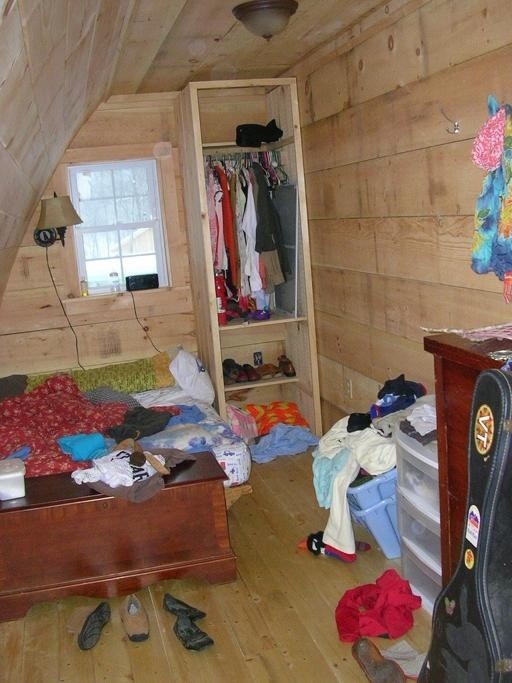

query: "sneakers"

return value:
[221, 353, 296, 385]
[119, 594, 149, 642]
[172, 615, 214, 652]
[163, 593, 201, 620]
[77, 602, 111, 651]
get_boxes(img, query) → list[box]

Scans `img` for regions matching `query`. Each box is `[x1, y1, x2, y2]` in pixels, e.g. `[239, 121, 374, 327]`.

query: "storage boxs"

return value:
[346, 466, 397, 560]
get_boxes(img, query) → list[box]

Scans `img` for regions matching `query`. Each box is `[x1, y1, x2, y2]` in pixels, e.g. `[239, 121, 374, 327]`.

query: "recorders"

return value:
[126, 273, 160, 290]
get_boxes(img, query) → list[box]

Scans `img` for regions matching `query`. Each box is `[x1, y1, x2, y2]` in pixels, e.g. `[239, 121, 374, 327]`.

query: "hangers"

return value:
[206, 148, 288, 193]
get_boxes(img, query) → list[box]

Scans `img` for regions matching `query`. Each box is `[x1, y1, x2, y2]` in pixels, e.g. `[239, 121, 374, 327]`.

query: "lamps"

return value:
[231, 0, 299, 41]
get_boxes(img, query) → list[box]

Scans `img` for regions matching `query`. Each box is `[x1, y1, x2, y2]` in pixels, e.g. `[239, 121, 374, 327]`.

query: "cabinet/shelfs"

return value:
[393, 323, 512, 617]
[172, 76, 323, 447]
[1, 451, 237, 621]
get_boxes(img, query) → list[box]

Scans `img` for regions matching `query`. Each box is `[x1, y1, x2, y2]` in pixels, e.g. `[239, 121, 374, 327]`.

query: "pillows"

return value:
[22, 350, 176, 394]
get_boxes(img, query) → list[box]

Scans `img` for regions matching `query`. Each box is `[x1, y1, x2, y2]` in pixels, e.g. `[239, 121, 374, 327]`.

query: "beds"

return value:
[0, 347, 253, 512]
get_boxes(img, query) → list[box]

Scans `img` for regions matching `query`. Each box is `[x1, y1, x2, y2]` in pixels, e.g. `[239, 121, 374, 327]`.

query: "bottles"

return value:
[80, 279, 89, 298]
[109, 272, 121, 292]
